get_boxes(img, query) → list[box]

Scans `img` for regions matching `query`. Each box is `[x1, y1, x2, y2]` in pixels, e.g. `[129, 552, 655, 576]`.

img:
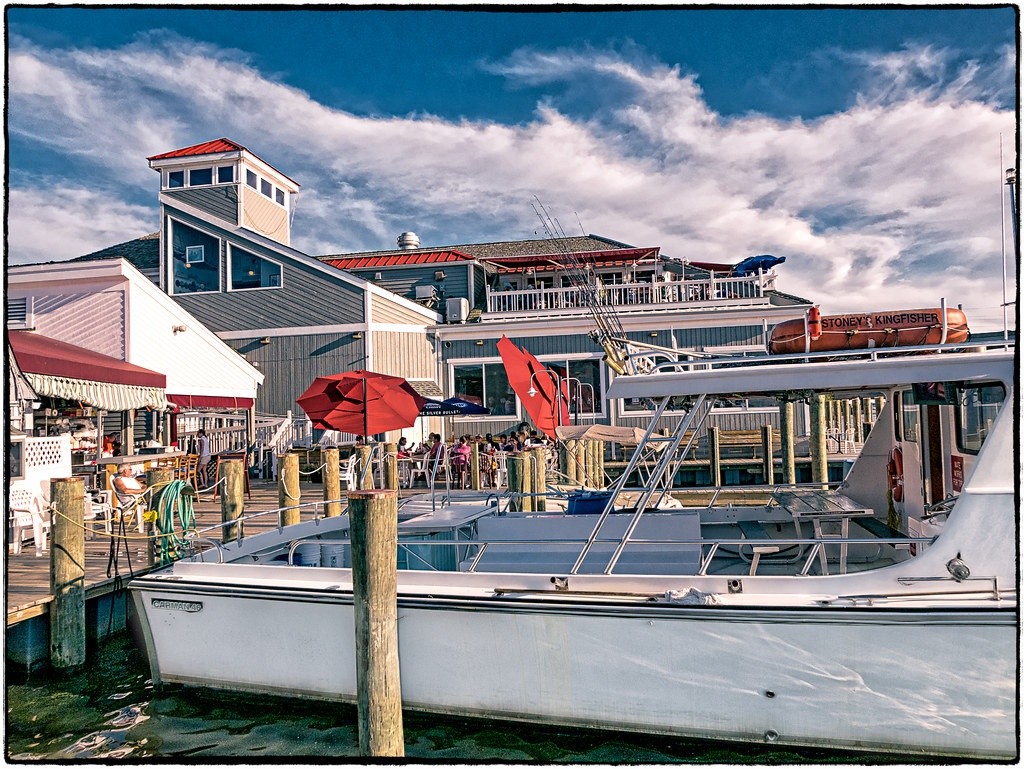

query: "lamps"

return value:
[526, 266, 537, 288]
[682, 256, 691, 280]
[172, 326, 187, 333]
[183, 263, 191, 269]
[584, 262, 590, 286]
[631, 259, 638, 283]
[476, 340, 484, 344]
[260, 336, 270, 344]
[650, 332, 657, 337]
[352, 332, 362, 339]
[434, 270, 447, 281]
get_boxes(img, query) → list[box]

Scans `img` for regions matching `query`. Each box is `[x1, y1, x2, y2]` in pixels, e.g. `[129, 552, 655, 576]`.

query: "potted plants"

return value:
[302, 463, 322, 483]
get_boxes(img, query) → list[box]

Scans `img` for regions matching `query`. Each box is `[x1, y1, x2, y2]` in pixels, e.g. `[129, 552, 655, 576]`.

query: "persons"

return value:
[499, 286, 512, 310]
[690, 283, 726, 300]
[102, 433, 120, 458]
[114, 462, 147, 508]
[658, 276, 666, 302]
[422, 432, 444, 481]
[567, 280, 584, 307]
[525, 284, 558, 309]
[451, 424, 558, 488]
[397, 438, 415, 484]
[350, 434, 365, 490]
[197, 429, 211, 488]
[602, 279, 638, 304]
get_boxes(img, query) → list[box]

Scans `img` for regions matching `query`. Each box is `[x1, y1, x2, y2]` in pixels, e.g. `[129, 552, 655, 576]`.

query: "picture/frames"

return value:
[186, 245, 204, 263]
[269, 274, 281, 286]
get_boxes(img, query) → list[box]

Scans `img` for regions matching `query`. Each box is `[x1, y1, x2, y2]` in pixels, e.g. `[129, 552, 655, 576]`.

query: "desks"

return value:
[96, 451, 187, 523]
[769, 489, 874, 565]
[397, 505, 497, 572]
[826, 433, 844, 454]
[397, 455, 424, 488]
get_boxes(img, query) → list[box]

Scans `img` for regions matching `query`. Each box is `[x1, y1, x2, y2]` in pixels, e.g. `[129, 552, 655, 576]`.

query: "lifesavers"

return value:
[887, 447, 904, 501]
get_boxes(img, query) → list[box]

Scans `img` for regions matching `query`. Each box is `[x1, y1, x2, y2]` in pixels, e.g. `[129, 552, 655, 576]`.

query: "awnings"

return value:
[6, 331, 167, 412]
[166, 394, 254, 409]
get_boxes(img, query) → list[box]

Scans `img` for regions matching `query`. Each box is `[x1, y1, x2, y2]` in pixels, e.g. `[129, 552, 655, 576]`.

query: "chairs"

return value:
[373, 447, 509, 490]
[8, 454, 200, 557]
[825, 428, 856, 454]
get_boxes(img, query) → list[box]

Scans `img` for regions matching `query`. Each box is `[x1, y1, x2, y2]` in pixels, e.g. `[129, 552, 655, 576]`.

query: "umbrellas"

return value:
[496, 334, 571, 440]
[295, 370, 425, 444]
[417, 396, 466, 444]
[442, 396, 492, 447]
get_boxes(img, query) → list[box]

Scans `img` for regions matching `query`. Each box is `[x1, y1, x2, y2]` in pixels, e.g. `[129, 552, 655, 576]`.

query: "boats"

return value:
[129, 298, 1017, 762]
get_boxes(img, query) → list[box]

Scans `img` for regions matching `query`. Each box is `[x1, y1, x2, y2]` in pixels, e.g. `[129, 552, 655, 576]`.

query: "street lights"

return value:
[527, 370, 595, 426]
[1005, 168, 1016, 250]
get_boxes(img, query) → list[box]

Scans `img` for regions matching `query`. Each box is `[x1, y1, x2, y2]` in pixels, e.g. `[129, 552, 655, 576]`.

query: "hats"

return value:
[197, 429, 206, 434]
[486, 433, 492, 439]
[498, 434, 507, 439]
[355, 435, 363, 440]
[475, 434, 483, 438]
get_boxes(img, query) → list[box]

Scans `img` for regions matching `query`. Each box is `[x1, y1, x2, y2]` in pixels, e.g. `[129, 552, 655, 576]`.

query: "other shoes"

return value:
[204, 485, 208, 488]
[198, 484, 205, 488]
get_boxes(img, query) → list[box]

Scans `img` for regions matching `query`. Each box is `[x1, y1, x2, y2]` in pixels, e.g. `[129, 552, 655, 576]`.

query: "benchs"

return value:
[619, 427, 699, 463]
[458, 513, 703, 577]
[736, 521, 779, 564]
[851, 516, 911, 564]
[718, 429, 783, 458]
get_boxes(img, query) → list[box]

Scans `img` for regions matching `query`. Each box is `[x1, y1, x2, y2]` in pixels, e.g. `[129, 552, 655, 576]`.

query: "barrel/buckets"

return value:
[567, 495, 614, 514]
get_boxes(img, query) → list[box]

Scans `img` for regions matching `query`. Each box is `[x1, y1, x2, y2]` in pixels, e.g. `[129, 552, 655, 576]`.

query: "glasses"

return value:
[112, 435, 117, 441]
[126, 468, 132, 470]
